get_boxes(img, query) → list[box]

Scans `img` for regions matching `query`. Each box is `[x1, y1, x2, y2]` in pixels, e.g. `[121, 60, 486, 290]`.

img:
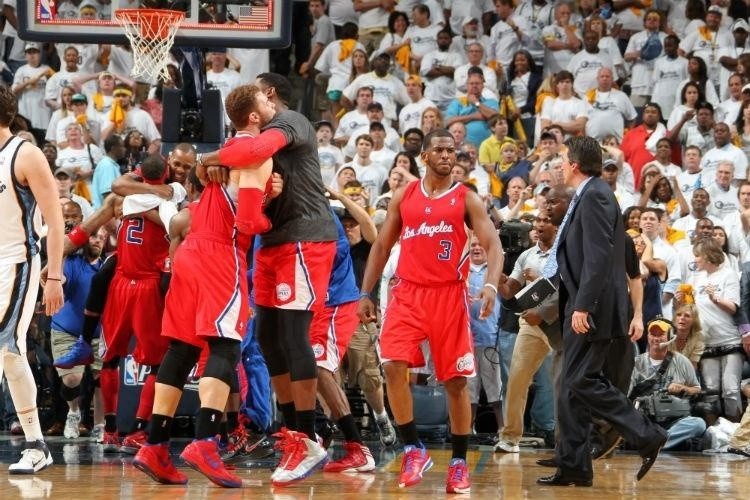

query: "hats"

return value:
[462, 15, 479, 25]
[640, 160, 665, 176]
[648, 320, 671, 333]
[99, 71, 115, 80]
[375, 50, 391, 59]
[316, 119, 332, 128]
[707, 5, 723, 15]
[534, 183, 550, 194]
[731, 18, 750, 34]
[603, 159, 618, 169]
[23, 42, 40, 53]
[71, 93, 88, 105]
[639, 33, 662, 60]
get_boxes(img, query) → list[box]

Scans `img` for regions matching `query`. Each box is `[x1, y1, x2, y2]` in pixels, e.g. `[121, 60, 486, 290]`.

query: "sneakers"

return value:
[399, 441, 433, 488]
[322, 418, 376, 473]
[271, 428, 330, 486]
[446, 457, 471, 493]
[7, 441, 53, 473]
[47, 422, 63, 434]
[64, 411, 89, 439]
[376, 413, 397, 448]
[178, 436, 241, 488]
[494, 428, 520, 452]
[10, 421, 22, 433]
[132, 442, 187, 484]
[53, 334, 94, 368]
[93, 423, 148, 454]
[216, 422, 273, 463]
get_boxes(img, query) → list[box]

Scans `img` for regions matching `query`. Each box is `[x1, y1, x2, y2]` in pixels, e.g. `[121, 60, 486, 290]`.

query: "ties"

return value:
[544, 194, 578, 278]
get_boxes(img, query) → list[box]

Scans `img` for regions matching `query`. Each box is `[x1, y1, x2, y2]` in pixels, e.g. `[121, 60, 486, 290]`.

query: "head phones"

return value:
[646, 318, 678, 339]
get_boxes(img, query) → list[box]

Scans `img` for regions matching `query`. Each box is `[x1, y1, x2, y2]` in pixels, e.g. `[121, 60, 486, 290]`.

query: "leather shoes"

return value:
[536, 456, 561, 467]
[537, 469, 593, 487]
[637, 425, 669, 481]
[589, 425, 622, 460]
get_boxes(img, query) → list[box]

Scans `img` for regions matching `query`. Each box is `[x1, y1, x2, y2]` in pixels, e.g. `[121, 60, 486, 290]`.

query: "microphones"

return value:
[658, 338, 677, 349]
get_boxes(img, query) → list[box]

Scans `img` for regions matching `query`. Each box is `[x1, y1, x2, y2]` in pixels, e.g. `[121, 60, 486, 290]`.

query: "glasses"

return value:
[130, 134, 143, 138]
